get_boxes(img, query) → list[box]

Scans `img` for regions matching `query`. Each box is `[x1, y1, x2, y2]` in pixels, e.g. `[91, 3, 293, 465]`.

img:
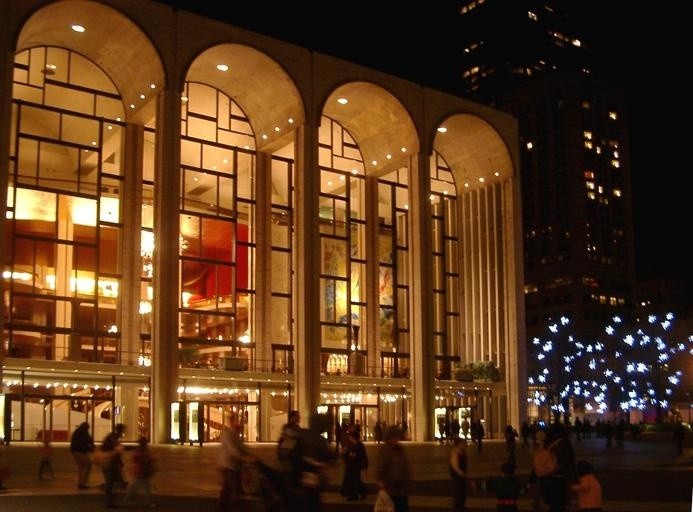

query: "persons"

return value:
[336, 369, 341, 376]
[216, 412, 692, 511]
[11, 343, 19, 357]
[70, 421, 157, 507]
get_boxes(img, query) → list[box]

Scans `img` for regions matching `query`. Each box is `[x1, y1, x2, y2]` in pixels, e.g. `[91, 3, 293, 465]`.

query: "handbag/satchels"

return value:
[533, 450, 559, 478]
